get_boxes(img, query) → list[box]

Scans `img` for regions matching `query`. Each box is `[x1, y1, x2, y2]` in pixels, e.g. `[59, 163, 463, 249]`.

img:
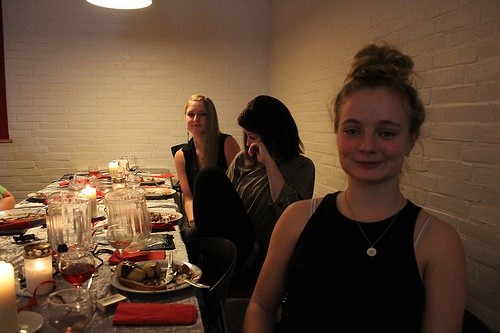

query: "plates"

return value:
[0, 208, 47, 224]
[27, 192, 57, 198]
[133, 176, 184, 223]
[17, 310, 44, 333]
[110, 259, 203, 294]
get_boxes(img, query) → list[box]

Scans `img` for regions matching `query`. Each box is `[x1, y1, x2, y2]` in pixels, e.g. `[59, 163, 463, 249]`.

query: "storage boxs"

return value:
[96, 294, 128, 314]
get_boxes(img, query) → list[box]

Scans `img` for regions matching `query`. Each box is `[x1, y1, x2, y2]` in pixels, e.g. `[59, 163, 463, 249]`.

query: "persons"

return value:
[223, 96, 315, 261]
[174, 95, 242, 225]
[243, 43, 466, 333]
[0, 185, 16, 212]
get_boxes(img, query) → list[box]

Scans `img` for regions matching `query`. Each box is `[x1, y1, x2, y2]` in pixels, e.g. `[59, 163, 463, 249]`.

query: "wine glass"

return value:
[58, 250, 96, 303]
[106, 224, 137, 272]
[69, 159, 101, 196]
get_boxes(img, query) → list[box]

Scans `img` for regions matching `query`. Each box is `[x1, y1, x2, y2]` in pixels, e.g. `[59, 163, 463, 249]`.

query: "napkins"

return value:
[151, 172, 175, 179]
[108, 250, 167, 264]
[97, 174, 111, 179]
[0, 219, 31, 229]
[110, 300, 199, 326]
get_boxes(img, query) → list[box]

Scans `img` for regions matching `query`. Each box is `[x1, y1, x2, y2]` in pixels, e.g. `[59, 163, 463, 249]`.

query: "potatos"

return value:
[116, 261, 161, 282]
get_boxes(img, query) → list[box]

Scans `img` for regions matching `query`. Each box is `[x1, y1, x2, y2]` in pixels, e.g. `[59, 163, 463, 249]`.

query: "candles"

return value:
[0, 260, 18, 333]
[80, 183, 98, 217]
[109, 161, 118, 176]
[24, 257, 52, 296]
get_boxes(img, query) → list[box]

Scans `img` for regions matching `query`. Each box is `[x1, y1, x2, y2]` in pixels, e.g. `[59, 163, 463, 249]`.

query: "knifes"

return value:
[166, 253, 173, 284]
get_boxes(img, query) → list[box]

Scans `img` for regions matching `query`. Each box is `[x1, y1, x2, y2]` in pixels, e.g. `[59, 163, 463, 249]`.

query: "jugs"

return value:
[46, 190, 94, 261]
[104, 186, 152, 253]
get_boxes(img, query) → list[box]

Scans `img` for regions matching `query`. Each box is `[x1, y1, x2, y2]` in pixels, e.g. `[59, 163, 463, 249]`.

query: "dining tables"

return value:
[0, 177, 205, 333]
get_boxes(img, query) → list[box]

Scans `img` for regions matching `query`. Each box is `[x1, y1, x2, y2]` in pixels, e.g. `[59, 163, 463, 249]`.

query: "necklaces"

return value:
[345, 191, 404, 256]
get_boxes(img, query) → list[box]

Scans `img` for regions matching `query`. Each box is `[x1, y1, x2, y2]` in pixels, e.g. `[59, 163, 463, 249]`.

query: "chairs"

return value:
[184, 234, 238, 333]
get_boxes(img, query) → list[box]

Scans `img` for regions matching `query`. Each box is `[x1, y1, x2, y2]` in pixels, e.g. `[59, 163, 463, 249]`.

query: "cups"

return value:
[42, 289, 93, 333]
[108, 155, 139, 189]
